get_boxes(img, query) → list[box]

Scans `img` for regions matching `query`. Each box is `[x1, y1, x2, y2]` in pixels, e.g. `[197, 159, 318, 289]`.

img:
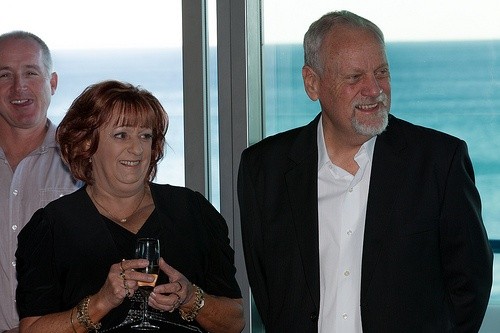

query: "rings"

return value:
[120, 262, 130, 296]
[169, 282, 182, 313]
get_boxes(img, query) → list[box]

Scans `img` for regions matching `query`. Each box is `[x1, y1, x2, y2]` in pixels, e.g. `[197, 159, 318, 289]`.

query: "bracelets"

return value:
[179, 284, 204, 322]
[71, 307, 77, 333]
[76, 296, 102, 331]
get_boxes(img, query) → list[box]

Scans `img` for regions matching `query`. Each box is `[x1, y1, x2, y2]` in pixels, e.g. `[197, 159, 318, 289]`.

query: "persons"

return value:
[15, 80, 245, 333]
[236, 10, 494, 333]
[0, 31, 84, 333]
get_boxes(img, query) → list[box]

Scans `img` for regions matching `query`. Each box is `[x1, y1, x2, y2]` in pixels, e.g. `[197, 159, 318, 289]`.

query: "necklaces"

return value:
[92, 182, 145, 223]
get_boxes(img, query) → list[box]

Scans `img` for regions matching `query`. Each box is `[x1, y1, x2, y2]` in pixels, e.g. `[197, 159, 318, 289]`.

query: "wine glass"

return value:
[130, 237, 160, 331]
